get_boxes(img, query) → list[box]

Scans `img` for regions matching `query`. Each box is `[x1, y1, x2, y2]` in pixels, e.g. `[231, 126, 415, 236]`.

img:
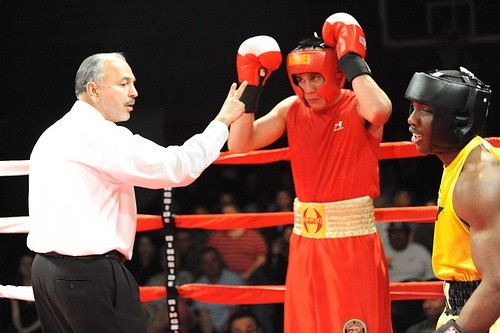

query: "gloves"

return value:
[321, 11, 376, 91]
[233, 34, 283, 114]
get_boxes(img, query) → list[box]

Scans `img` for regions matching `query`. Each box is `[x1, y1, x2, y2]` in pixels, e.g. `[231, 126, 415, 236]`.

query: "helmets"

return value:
[285, 31, 348, 109]
[403, 64, 493, 153]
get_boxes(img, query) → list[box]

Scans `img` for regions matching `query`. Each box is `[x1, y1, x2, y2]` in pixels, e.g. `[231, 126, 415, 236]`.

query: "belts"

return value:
[43, 250, 127, 265]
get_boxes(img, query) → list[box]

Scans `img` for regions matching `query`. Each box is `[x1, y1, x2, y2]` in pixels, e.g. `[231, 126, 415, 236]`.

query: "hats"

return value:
[387, 222, 410, 232]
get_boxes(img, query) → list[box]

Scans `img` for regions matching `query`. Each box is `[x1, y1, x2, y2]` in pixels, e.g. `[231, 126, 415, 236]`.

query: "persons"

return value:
[231, 13, 392, 333]
[405, 66, 500, 333]
[30, 53, 250, 333]
[11, 187, 445, 333]
